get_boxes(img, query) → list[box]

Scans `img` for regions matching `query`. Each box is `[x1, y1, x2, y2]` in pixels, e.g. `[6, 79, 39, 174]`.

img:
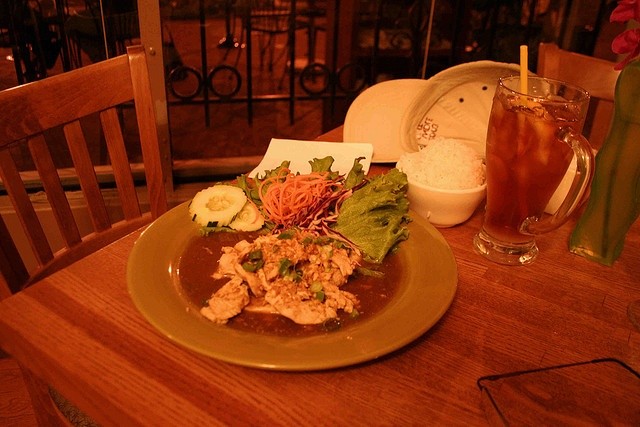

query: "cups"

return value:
[473, 75, 595, 266]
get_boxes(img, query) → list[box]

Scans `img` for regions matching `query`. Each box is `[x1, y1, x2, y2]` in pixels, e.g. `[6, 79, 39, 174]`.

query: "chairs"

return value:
[0, 44, 169, 427]
[229, 0, 305, 89]
[93, 4, 141, 58]
[537, 40, 621, 150]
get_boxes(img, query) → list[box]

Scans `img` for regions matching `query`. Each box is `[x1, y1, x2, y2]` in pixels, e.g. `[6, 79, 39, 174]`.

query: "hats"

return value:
[343, 60, 553, 163]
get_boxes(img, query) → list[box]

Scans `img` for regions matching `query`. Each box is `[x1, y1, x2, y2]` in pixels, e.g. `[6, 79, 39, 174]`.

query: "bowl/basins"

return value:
[396, 154, 487, 226]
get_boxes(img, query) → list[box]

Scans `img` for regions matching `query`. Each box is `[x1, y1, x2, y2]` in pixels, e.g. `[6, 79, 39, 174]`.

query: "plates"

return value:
[127, 186, 459, 372]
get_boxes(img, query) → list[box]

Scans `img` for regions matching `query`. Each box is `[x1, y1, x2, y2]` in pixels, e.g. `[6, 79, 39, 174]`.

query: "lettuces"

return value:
[235, 155, 412, 262]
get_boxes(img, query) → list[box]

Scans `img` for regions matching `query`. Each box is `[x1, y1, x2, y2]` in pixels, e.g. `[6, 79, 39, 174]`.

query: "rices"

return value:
[400, 138, 485, 188]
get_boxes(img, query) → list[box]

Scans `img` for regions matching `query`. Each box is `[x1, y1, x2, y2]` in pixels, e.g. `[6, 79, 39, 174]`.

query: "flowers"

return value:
[610, 0, 640, 72]
